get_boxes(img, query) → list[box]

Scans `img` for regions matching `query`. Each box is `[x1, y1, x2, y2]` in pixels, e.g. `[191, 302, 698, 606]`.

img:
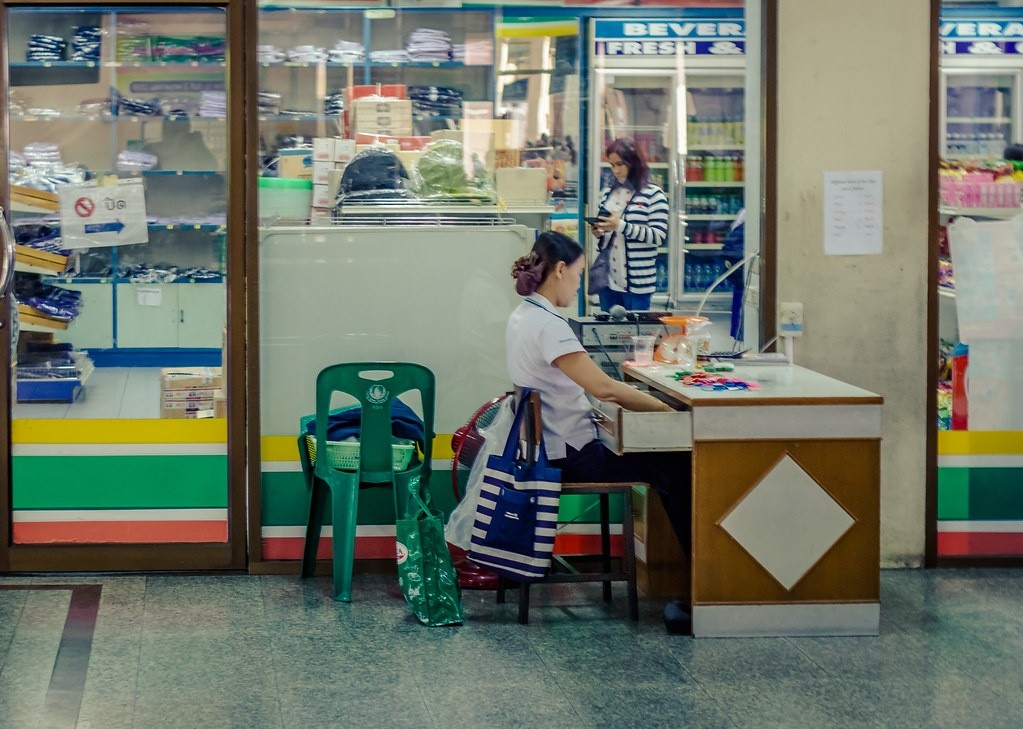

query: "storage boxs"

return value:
[159, 366, 222, 419]
[257, 177, 315, 226]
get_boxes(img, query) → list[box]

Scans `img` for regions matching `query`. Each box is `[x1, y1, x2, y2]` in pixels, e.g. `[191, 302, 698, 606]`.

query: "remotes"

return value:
[594, 311, 611, 321]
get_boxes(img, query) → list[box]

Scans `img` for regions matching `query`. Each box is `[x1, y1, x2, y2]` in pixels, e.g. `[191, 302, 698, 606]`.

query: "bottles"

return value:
[685, 264, 735, 291]
[602, 134, 665, 192]
[685, 193, 742, 214]
[686, 155, 744, 182]
[687, 113, 744, 146]
[947, 133, 1006, 155]
[655, 264, 667, 292]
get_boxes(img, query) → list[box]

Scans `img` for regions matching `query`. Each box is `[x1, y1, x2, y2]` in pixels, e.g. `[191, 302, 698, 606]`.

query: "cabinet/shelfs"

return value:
[4, 4, 583, 372]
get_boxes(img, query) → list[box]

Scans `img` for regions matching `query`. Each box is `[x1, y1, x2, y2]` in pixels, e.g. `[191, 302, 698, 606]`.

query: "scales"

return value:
[654, 315, 710, 363]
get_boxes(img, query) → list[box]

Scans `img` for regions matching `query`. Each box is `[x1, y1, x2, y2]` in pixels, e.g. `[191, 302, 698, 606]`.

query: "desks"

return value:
[618, 352, 885, 638]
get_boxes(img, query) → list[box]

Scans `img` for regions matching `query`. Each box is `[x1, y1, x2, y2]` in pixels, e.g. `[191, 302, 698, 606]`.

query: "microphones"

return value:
[610, 305, 626, 320]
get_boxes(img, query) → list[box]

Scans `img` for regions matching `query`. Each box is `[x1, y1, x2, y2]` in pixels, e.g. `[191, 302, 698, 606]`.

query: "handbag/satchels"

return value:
[443, 388, 562, 579]
[394, 475, 464, 626]
[588, 249, 610, 294]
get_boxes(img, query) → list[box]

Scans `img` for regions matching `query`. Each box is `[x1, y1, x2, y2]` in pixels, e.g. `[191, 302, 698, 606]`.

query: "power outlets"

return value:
[777, 301, 804, 337]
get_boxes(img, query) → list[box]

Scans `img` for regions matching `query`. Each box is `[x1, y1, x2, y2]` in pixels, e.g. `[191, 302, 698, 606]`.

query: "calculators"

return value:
[709, 348, 752, 358]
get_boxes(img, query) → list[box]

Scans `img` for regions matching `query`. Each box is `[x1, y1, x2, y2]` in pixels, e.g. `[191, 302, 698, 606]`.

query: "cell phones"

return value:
[583, 217, 606, 229]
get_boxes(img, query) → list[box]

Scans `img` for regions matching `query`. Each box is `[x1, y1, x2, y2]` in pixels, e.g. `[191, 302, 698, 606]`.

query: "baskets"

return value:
[305, 435, 414, 471]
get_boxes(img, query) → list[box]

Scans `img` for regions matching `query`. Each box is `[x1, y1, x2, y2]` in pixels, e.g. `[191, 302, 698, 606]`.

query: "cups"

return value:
[630, 336, 656, 365]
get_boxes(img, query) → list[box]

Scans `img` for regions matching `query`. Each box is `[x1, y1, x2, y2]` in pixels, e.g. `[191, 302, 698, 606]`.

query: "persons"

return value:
[590, 137, 668, 311]
[142, 110, 219, 266]
[1004, 143, 1023, 161]
[509, 229, 693, 622]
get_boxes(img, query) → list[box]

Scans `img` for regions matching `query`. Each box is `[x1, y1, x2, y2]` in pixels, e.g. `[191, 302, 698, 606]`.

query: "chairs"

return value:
[296, 361, 436, 601]
[496, 383, 650, 623]
